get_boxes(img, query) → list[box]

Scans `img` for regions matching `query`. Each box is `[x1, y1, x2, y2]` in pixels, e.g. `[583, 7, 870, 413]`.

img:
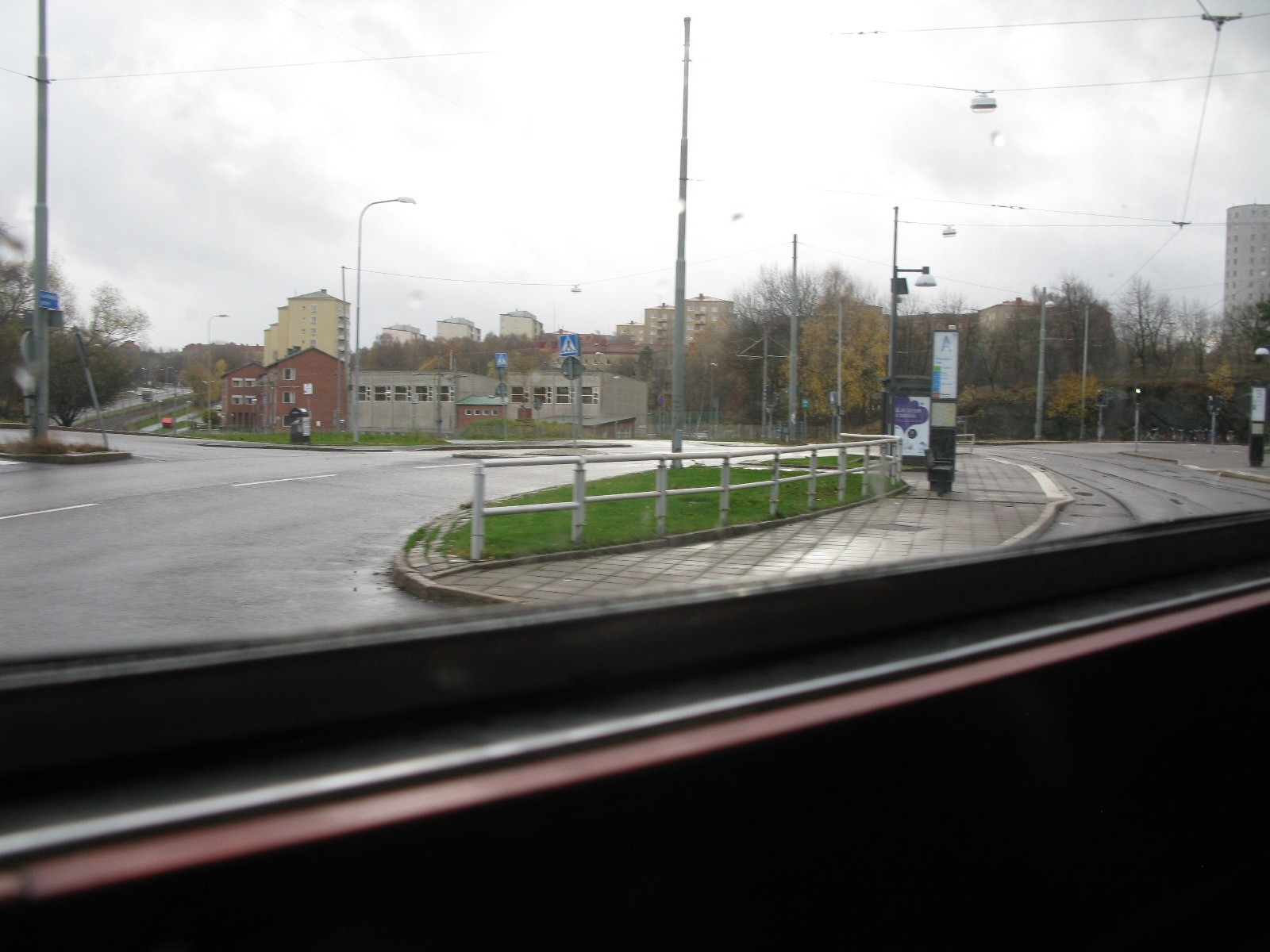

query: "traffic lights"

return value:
[1136, 384, 1142, 404]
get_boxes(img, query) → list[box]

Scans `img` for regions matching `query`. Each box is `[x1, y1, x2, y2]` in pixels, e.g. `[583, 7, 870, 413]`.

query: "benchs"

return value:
[923, 447, 956, 496]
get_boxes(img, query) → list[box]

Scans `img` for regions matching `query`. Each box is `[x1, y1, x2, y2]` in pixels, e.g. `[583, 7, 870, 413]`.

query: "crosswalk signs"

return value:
[559, 335, 579, 356]
[496, 353, 508, 367]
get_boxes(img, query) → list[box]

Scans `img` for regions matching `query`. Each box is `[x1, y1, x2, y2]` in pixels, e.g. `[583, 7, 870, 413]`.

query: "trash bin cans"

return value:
[289, 407, 310, 444]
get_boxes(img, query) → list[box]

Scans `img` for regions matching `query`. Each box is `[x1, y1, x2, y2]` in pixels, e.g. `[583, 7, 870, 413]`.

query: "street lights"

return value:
[205, 314, 231, 431]
[886, 267, 937, 435]
[1033, 298, 1055, 441]
[353, 197, 416, 444]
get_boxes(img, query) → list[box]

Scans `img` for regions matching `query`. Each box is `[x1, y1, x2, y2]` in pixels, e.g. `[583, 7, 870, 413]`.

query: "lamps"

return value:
[969, 89, 997, 113]
[570, 284, 581, 292]
[942, 224, 956, 238]
